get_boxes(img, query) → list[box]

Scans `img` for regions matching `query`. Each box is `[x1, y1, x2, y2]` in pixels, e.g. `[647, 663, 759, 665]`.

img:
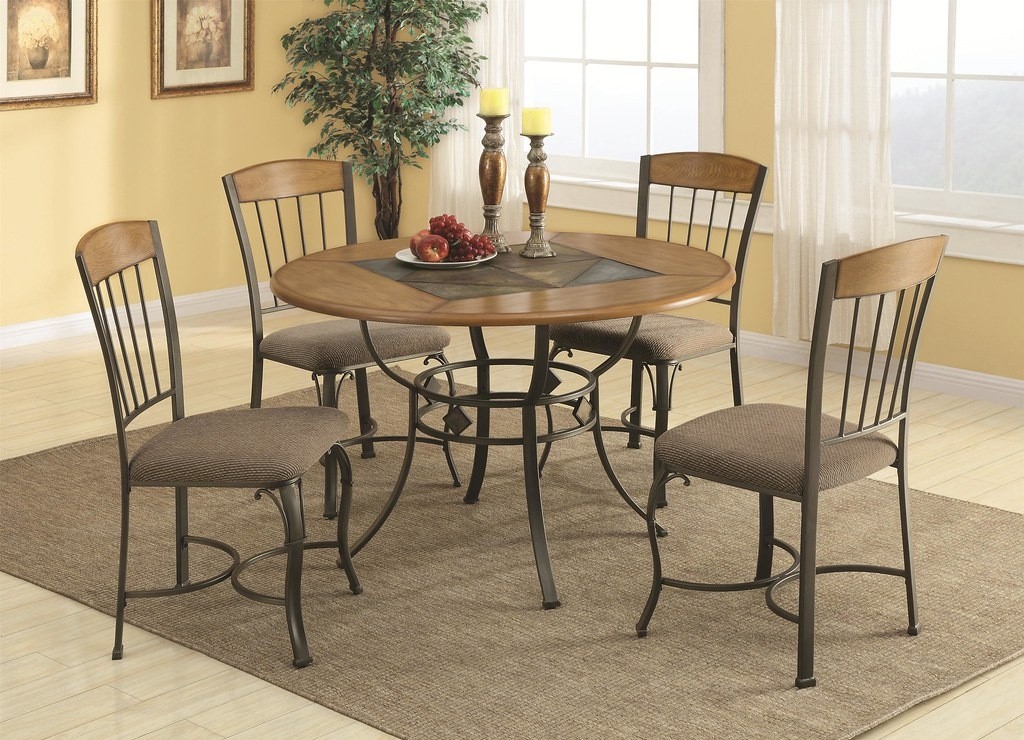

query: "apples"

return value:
[409, 230, 449, 262]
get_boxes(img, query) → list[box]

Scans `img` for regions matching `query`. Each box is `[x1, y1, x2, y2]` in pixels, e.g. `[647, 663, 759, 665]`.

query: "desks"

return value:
[269, 231, 738, 607]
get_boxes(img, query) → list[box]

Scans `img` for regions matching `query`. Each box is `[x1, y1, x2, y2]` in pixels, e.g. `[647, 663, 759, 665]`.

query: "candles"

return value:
[522, 109, 552, 135]
[479, 87, 508, 115]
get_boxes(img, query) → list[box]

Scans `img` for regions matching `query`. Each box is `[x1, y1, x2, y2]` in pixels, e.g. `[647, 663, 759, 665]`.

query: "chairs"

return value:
[74, 219, 362, 671]
[538, 153, 769, 507]
[636, 232, 950, 689]
[222, 159, 462, 520]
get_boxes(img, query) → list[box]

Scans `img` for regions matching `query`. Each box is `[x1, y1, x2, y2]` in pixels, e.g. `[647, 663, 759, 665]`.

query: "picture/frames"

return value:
[149, 0, 256, 101]
[0, 0, 99, 112]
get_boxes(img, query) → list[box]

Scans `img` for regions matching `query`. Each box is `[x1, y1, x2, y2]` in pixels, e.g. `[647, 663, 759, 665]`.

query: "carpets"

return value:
[0, 365, 1024, 740]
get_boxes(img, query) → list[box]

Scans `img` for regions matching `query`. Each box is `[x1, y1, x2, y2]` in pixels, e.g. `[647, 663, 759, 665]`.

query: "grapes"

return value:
[428, 213, 496, 262]
